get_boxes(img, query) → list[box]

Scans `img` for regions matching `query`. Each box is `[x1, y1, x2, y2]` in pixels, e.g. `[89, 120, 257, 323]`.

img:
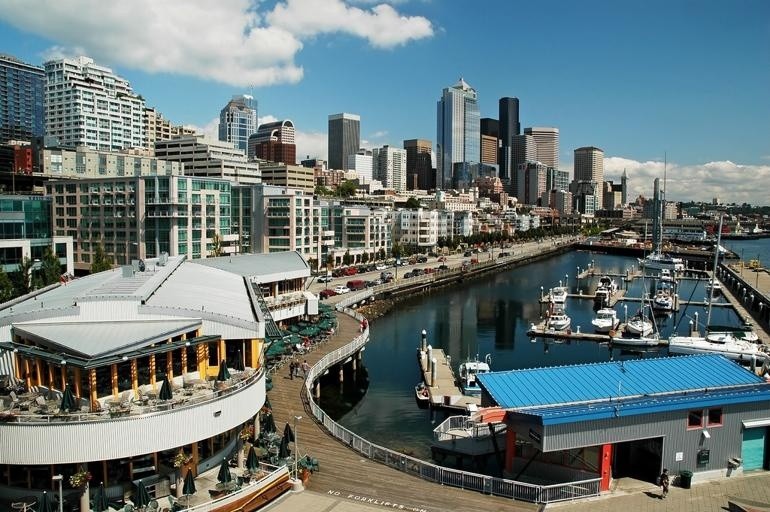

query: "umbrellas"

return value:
[266, 374, 272, 381]
[284, 421, 295, 443]
[93, 482, 109, 512]
[218, 359, 231, 380]
[246, 443, 260, 469]
[159, 375, 172, 400]
[62, 383, 76, 411]
[134, 480, 151, 508]
[217, 457, 232, 487]
[266, 382, 273, 391]
[39, 491, 54, 511]
[261, 301, 337, 360]
[265, 394, 273, 410]
[280, 434, 291, 457]
[264, 411, 277, 433]
[182, 467, 197, 508]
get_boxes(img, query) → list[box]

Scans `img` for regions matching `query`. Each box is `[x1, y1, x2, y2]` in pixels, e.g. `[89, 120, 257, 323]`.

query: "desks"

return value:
[12, 495, 38, 512]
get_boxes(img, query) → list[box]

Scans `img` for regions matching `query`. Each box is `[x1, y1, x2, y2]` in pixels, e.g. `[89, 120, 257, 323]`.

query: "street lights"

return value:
[294, 415, 302, 481]
[52, 474, 64, 512]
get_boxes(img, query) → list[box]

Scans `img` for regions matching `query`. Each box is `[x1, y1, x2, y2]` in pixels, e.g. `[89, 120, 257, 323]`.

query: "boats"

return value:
[414, 381, 430, 401]
[593, 276, 619, 303]
[458, 352, 494, 396]
[705, 277, 721, 292]
[546, 311, 571, 331]
[636, 251, 685, 271]
[736, 316, 759, 341]
[652, 268, 678, 311]
[591, 308, 620, 333]
[626, 309, 654, 337]
[550, 286, 568, 303]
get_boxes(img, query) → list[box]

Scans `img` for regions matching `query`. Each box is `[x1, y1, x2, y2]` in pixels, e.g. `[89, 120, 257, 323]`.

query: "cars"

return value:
[404, 265, 450, 278]
[464, 248, 482, 257]
[400, 255, 427, 265]
[317, 259, 394, 299]
[438, 257, 447, 262]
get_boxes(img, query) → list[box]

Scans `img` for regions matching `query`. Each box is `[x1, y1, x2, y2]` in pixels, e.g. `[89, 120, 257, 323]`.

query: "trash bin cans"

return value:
[680, 471, 693, 488]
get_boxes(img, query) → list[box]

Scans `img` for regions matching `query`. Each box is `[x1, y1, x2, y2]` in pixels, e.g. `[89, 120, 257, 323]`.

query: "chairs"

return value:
[0, 368, 254, 423]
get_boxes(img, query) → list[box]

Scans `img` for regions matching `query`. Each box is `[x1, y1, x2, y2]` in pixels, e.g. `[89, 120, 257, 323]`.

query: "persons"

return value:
[295, 358, 301, 375]
[302, 359, 311, 374]
[289, 361, 295, 380]
[659, 468, 671, 501]
